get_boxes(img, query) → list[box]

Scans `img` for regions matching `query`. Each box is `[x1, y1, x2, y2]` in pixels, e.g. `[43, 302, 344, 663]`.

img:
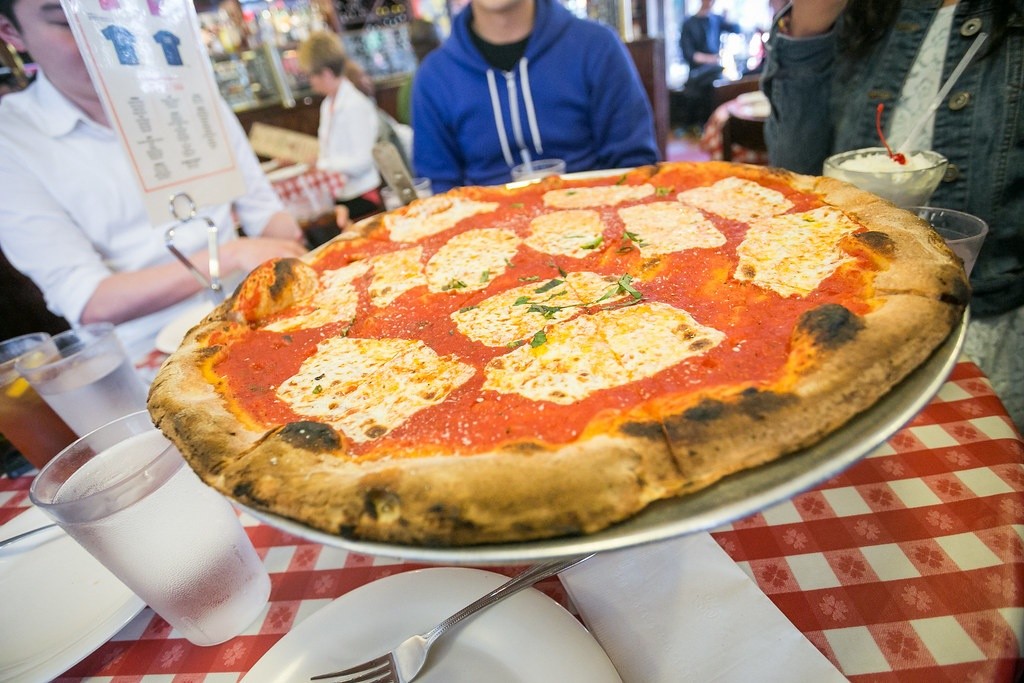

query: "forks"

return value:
[307, 549, 599, 683]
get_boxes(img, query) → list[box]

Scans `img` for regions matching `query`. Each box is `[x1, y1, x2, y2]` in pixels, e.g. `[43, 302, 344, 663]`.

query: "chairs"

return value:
[623, 38, 670, 162]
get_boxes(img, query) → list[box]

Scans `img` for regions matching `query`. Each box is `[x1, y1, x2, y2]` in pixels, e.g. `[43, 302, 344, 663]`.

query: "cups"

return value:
[510, 159, 566, 184]
[0, 331, 78, 473]
[820, 148, 950, 208]
[379, 176, 434, 214]
[898, 206, 988, 280]
[28, 409, 272, 648]
[15, 320, 147, 438]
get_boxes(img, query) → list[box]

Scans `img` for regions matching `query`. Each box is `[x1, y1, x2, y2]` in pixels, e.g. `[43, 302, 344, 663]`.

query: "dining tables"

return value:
[266, 160, 335, 230]
[0, 357, 1022, 682]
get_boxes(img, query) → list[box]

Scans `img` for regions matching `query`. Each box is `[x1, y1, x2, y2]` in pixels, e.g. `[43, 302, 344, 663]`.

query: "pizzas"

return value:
[149, 160, 973, 543]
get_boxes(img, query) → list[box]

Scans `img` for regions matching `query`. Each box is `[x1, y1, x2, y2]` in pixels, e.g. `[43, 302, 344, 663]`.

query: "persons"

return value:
[678, 0, 744, 89]
[0, 1, 311, 330]
[297, 30, 385, 208]
[757, 0, 1023, 435]
[408, 0, 663, 197]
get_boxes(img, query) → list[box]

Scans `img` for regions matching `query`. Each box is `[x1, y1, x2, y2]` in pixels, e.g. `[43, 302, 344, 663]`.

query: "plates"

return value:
[236, 566, 625, 683]
[1, 504, 149, 682]
[220, 169, 971, 568]
[156, 293, 238, 354]
